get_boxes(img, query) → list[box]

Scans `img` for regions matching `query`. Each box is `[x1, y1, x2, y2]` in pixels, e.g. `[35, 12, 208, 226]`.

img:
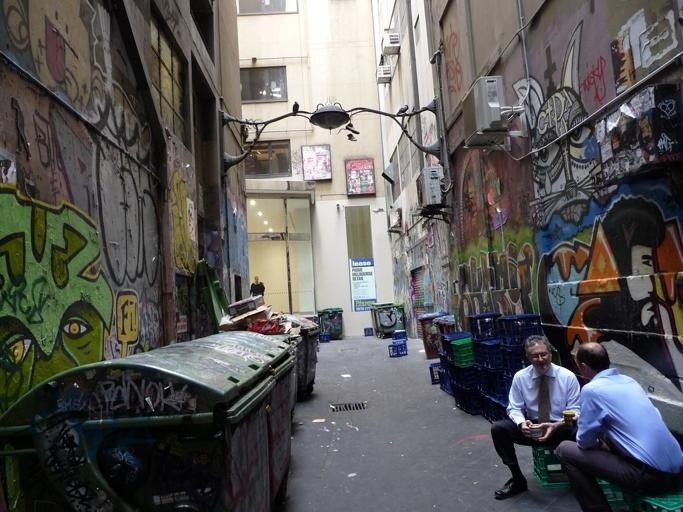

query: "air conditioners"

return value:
[415, 167, 442, 207]
[460, 75, 509, 139]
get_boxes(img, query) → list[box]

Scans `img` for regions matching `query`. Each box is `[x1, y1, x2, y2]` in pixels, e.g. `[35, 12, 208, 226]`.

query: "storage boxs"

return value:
[428, 311, 543, 425]
[393, 339, 407, 351]
[641, 491, 683, 512]
[599, 479, 624, 503]
[392, 332, 409, 341]
[388, 343, 407, 357]
[320, 333, 330, 343]
[364, 328, 373, 336]
[530, 446, 570, 488]
[227, 295, 266, 317]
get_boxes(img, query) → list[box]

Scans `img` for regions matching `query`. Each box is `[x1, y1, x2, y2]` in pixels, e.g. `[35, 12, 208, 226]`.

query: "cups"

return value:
[561, 411, 576, 427]
[529, 424, 542, 441]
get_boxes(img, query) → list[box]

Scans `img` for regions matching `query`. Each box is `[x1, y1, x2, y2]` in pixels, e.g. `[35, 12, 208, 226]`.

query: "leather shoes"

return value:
[495, 476, 528, 500]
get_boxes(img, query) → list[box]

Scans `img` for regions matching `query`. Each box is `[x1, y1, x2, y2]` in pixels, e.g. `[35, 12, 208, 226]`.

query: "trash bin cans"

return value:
[416, 311, 447, 360]
[283, 315, 319, 402]
[317, 308, 343, 341]
[0, 347, 271, 512]
[370, 302, 406, 340]
[171, 331, 291, 508]
[433, 315, 456, 334]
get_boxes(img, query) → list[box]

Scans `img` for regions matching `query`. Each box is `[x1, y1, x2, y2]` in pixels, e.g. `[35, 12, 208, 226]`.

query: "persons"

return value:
[557, 341, 683, 512]
[250, 276, 265, 297]
[491, 335, 581, 500]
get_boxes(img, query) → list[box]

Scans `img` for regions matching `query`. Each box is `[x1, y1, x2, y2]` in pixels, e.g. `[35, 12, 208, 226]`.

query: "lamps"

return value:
[217, 97, 450, 193]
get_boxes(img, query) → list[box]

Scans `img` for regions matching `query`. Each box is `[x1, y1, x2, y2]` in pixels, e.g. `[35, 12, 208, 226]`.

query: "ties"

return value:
[538, 375, 551, 424]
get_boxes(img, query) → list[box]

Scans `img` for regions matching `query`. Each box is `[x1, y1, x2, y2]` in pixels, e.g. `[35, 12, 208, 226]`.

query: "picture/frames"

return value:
[344, 158, 377, 197]
[300, 143, 332, 181]
